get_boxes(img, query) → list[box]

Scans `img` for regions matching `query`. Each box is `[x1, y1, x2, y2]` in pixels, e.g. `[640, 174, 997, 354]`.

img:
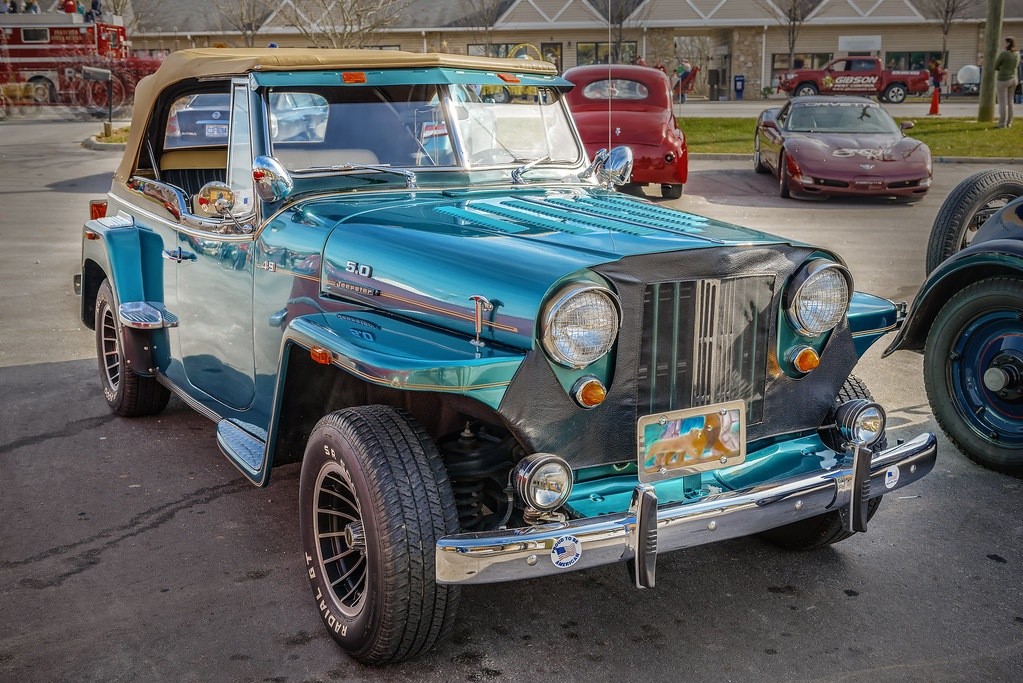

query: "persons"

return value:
[0, 0, 103, 22]
[979, 37, 1020, 128]
[629, 55, 690, 105]
[886, 59, 925, 70]
[932, 60, 947, 104]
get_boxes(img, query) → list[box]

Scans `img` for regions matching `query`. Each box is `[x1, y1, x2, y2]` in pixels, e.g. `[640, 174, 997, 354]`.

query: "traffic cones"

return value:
[926, 88, 942, 116]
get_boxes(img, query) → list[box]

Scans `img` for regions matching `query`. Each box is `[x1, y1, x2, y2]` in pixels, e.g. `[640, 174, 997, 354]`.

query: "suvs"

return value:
[74, 47, 941, 667]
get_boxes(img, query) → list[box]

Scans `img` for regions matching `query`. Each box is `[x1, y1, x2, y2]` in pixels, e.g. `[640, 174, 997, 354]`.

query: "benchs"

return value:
[160, 149, 380, 215]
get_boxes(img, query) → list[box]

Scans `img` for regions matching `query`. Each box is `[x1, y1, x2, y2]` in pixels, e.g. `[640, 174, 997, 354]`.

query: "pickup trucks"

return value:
[780, 56, 931, 105]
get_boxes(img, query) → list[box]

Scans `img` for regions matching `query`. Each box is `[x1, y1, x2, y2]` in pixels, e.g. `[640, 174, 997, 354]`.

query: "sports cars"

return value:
[752, 93, 933, 203]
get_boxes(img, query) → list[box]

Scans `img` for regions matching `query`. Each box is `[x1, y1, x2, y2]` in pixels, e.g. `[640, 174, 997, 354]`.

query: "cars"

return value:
[559, 65, 687, 199]
